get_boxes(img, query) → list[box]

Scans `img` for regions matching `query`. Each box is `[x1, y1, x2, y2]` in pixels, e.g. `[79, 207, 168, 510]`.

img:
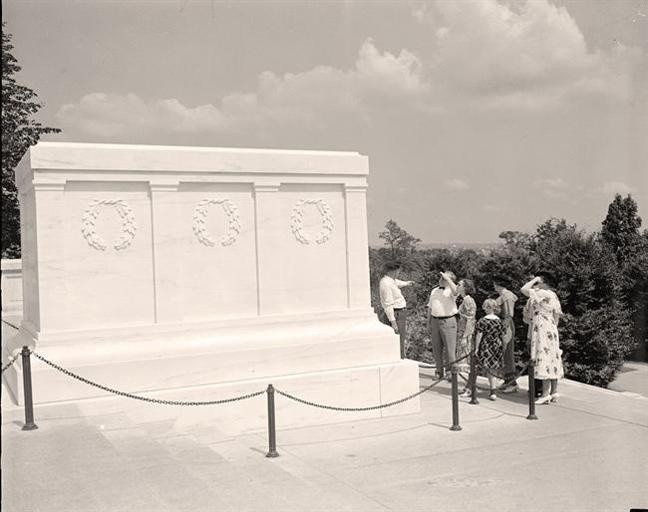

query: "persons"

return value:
[519, 271, 563, 405]
[457, 298, 506, 400]
[453, 279, 477, 373]
[489, 276, 520, 393]
[425, 269, 459, 383]
[521, 276, 549, 398]
[378, 262, 415, 359]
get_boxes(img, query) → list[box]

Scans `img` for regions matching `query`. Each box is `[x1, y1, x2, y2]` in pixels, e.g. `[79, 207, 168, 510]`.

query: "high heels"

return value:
[534, 394, 559, 404]
[499, 384, 518, 393]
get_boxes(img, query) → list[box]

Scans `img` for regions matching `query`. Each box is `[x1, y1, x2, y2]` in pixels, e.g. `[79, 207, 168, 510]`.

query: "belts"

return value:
[432, 315, 455, 319]
[394, 308, 406, 311]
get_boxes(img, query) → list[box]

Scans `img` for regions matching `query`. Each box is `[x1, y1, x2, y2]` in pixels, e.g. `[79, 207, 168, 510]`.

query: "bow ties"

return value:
[440, 287, 445, 289]
